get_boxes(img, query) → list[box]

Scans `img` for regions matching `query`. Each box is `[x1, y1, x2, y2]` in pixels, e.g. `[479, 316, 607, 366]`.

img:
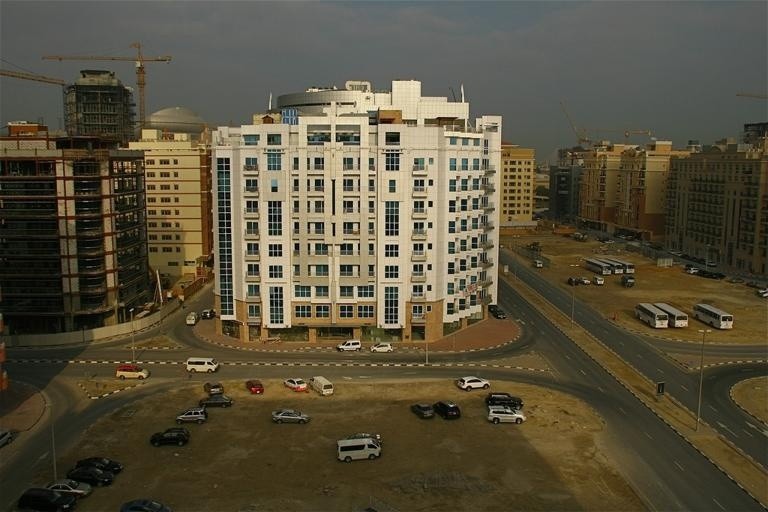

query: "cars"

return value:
[0, 430, 13, 447]
[668, 248, 703, 264]
[272, 407, 311, 425]
[370, 342, 394, 353]
[410, 401, 461, 420]
[199, 380, 233, 409]
[730, 277, 744, 284]
[346, 431, 384, 445]
[246, 379, 265, 394]
[685, 261, 725, 280]
[491, 306, 506, 319]
[567, 275, 591, 285]
[120, 498, 172, 512]
[44, 455, 125, 499]
[283, 377, 309, 392]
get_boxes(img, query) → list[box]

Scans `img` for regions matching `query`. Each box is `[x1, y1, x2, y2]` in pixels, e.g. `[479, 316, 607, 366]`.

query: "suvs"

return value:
[486, 392, 527, 424]
[457, 376, 491, 392]
[18, 487, 79, 512]
[149, 406, 209, 447]
[201, 309, 215, 320]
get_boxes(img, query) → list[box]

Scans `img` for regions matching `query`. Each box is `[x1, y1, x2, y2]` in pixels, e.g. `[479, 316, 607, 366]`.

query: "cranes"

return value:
[40, 39, 174, 130]
[558, 98, 595, 145]
[737, 91, 768, 100]
[584, 121, 652, 145]
[0, 58, 71, 86]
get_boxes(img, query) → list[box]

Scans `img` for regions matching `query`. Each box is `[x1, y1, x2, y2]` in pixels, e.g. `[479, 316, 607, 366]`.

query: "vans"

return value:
[534, 259, 543, 269]
[309, 376, 335, 397]
[185, 311, 199, 326]
[336, 438, 383, 463]
[593, 276, 605, 286]
[335, 339, 363, 352]
[116, 365, 149, 380]
[186, 357, 220, 374]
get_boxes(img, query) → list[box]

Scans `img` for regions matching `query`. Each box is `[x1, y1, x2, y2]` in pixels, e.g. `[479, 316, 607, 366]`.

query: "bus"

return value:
[635, 301, 734, 331]
[584, 257, 636, 277]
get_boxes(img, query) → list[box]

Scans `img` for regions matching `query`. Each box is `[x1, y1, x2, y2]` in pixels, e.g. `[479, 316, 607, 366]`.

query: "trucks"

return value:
[620, 275, 635, 287]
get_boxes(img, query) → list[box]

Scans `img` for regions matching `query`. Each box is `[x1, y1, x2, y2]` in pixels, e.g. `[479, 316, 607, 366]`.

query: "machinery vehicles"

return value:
[527, 241, 544, 251]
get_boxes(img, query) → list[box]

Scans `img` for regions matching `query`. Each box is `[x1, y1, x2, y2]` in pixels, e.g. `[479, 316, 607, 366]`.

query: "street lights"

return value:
[696, 330, 712, 432]
[128, 308, 136, 362]
[513, 234, 520, 280]
